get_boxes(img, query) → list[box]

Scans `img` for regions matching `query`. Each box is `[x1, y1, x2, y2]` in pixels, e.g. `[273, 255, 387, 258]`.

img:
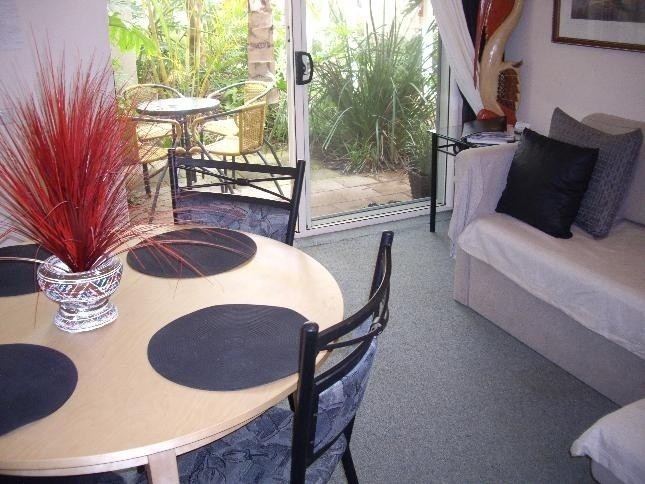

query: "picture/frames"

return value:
[551, 0, 645, 53]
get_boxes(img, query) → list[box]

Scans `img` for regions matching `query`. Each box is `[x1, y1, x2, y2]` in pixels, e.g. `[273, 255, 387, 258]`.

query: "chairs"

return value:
[196, 80, 283, 189]
[122, 83, 193, 197]
[190, 103, 286, 201]
[167, 148, 304, 246]
[177, 232, 397, 484]
[117, 118, 184, 227]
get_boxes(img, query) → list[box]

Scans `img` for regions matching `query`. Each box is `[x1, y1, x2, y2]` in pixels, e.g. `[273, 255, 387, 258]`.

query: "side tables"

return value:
[427, 124, 524, 233]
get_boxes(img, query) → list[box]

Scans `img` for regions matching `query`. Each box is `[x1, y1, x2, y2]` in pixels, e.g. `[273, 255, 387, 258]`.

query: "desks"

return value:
[0, 221, 345, 484]
[137, 96, 221, 188]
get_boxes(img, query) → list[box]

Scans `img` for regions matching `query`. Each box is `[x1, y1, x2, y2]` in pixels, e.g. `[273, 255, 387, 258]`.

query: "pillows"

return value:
[548, 108, 642, 239]
[495, 128, 599, 239]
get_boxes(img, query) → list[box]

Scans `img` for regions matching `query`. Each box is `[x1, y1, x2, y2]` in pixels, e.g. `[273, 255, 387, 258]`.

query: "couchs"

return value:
[452, 112, 645, 407]
[570, 399, 645, 482]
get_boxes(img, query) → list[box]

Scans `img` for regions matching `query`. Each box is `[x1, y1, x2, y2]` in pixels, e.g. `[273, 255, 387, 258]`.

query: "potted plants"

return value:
[0, 25, 251, 333]
[406, 120, 435, 199]
